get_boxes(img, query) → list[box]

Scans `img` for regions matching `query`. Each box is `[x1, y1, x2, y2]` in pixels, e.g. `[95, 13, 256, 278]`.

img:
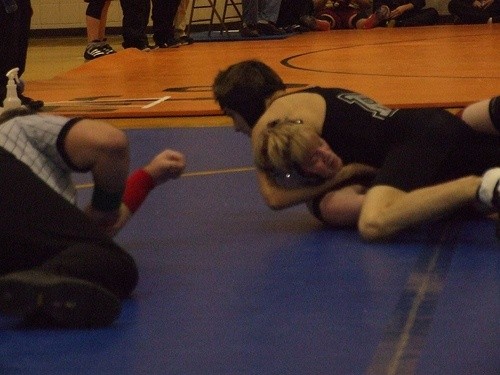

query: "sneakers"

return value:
[474, 164, 500, 211]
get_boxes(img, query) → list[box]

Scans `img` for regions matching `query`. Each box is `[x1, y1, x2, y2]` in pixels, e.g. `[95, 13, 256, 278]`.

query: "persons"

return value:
[210, 58, 500, 243]
[240, 0, 286, 38]
[120, 0, 159, 52]
[0, 108, 140, 332]
[254, 95, 500, 231]
[172, 0, 195, 45]
[152, 0, 182, 49]
[448, 0, 500, 24]
[298, 0, 390, 31]
[373, 0, 440, 28]
[0, 0, 43, 108]
[84, 0, 117, 59]
[80, 149, 189, 242]
[276, 0, 308, 33]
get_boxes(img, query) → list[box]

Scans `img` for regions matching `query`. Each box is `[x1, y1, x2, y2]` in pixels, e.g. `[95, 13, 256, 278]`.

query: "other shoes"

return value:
[176, 39, 188, 46]
[100, 44, 117, 55]
[146, 44, 159, 51]
[180, 36, 194, 44]
[0, 272, 122, 331]
[362, 4, 391, 30]
[239, 15, 307, 35]
[83, 46, 106, 60]
[122, 42, 151, 52]
[156, 40, 181, 48]
[19, 93, 44, 109]
[300, 14, 330, 30]
